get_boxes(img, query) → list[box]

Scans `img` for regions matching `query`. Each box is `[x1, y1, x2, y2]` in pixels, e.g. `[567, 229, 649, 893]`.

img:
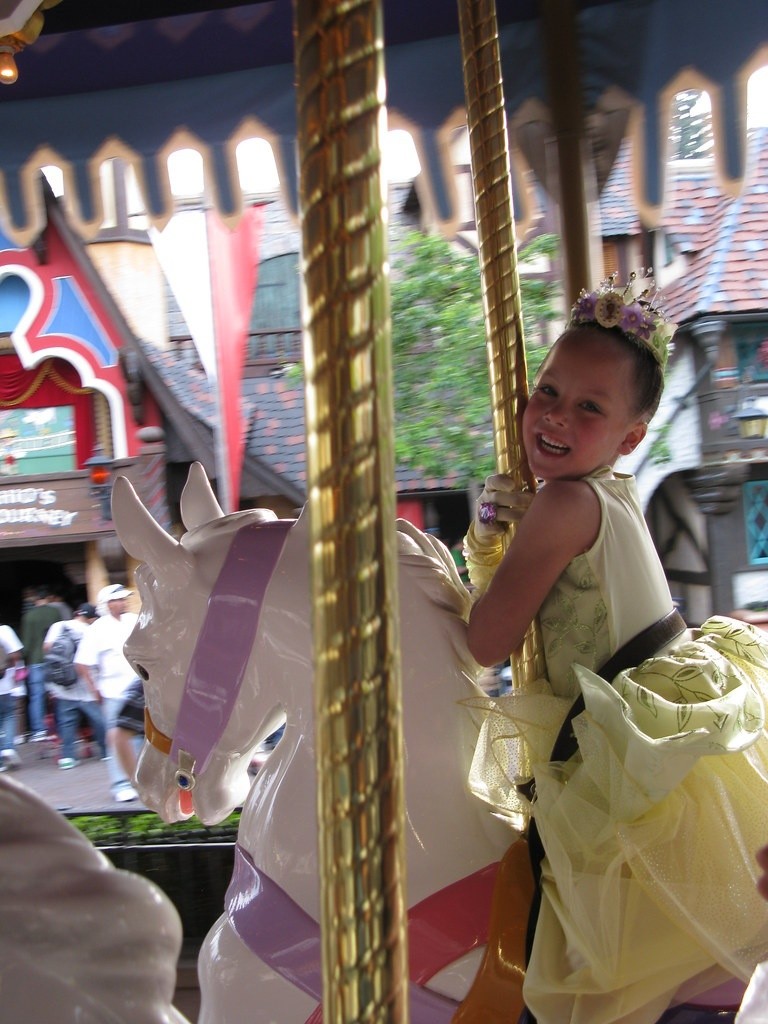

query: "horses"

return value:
[109, 460, 768, 1024]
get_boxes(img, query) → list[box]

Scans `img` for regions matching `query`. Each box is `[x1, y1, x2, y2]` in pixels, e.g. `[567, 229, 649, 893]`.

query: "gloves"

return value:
[473, 473, 535, 547]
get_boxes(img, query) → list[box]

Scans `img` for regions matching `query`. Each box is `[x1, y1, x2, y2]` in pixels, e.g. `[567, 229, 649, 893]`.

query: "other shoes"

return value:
[13, 734, 26, 746]
[111, 781, 139, 802]
[26, 730, 48, 743]
[0, 748, 24, 768]
[56, 757, 82, 769]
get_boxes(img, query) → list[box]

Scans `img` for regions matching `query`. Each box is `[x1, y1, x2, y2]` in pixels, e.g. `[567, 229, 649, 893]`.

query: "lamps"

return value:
[83, 445, 117, 521]
[723, 386, 767, 439]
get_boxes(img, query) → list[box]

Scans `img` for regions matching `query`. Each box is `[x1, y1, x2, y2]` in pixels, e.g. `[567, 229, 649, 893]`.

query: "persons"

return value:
[462, 270, 768, 1024]
[0, 584, 151, 803]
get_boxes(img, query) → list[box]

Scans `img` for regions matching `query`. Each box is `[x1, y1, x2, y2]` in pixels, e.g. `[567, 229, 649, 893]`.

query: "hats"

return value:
[73, 603, 99, 619]
[97, 584, 134, 604]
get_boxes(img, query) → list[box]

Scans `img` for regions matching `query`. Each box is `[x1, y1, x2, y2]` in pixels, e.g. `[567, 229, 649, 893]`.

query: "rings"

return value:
[480, 502, 497, 525]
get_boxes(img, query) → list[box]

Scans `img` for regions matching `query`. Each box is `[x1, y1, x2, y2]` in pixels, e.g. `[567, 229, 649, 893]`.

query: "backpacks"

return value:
[42, 625, 83, 687]
